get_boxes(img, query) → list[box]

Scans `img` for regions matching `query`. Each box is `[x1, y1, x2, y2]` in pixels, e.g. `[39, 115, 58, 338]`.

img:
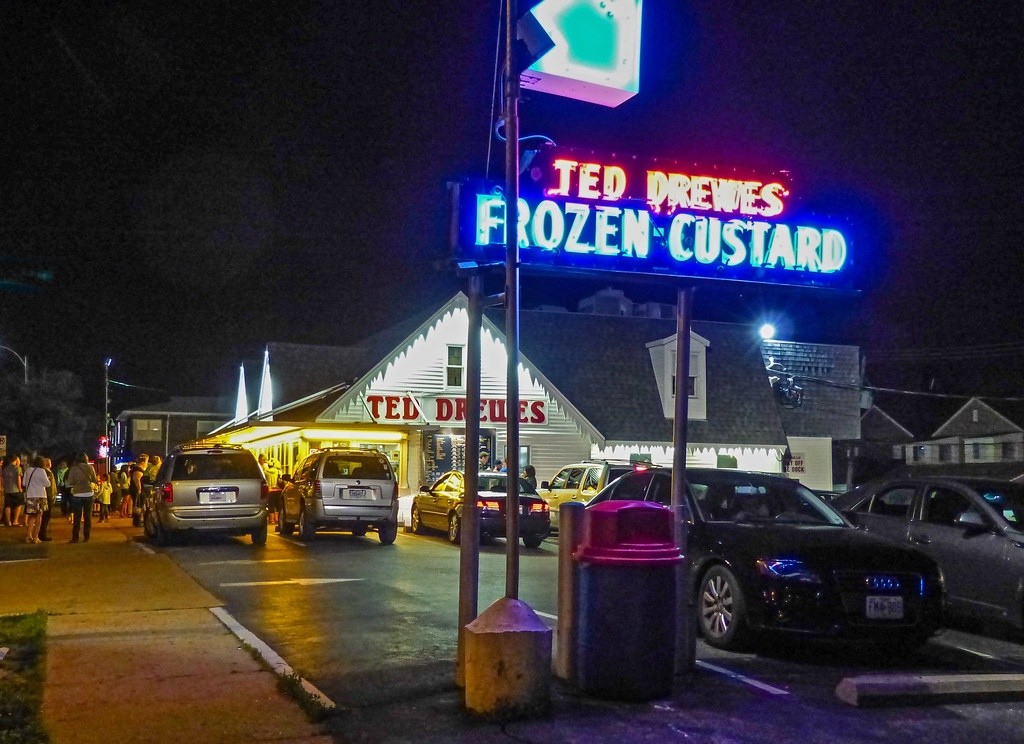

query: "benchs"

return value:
[325, 463, 380, 475]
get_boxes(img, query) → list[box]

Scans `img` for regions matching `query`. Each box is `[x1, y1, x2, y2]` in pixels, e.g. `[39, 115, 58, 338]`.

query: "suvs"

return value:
[537, 461, 663, 542]
[583, 467, 948, 656]
[139, 438, 269, 545]
[280, 447, 399, 543]
[411, 469, 551, 549]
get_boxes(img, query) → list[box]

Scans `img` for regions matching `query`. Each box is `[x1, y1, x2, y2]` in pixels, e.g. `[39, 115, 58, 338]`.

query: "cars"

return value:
[826, 460, 1024, 642]
[812, 490, 848, 503]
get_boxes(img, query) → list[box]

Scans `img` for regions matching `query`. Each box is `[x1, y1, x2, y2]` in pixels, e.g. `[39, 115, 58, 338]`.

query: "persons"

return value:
[479, 451, 537, 492]
[0, 452, 164, 544]
[257, 454, 293, 525]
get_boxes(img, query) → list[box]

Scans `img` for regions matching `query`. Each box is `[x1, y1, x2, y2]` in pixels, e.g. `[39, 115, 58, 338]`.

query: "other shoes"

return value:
[25, 536, 34, 543]
[33, 538, 42, 543]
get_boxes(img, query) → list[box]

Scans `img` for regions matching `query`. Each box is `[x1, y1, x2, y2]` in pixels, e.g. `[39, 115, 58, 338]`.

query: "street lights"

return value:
[104, 357, 112, 473]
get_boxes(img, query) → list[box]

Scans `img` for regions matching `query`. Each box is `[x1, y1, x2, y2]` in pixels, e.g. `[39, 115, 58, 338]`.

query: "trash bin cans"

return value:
[574, 501, 685, 703]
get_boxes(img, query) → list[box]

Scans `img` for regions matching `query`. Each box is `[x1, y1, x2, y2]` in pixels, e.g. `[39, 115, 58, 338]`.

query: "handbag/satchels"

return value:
[134, 506, 142, 516]
